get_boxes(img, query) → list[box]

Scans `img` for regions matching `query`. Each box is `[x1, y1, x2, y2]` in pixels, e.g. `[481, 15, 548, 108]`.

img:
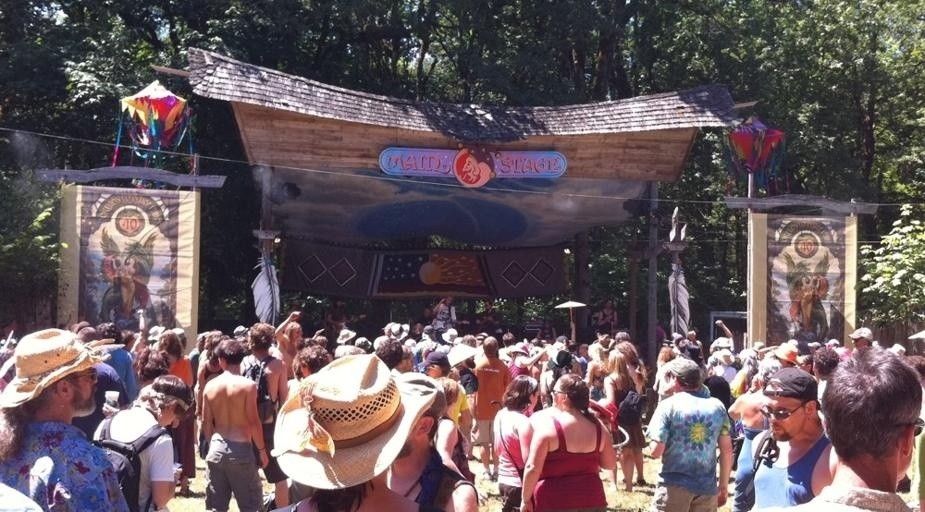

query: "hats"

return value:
[772, 342, 798, 366]
[511, 342, 529, 356]
[395, 371, 447, 420]
[355, 336, 373, 351]
[886, 343, 907, 358]
[336, 328, 357, 344]
[390, 322, 411, 341]
[146, 324, 166, 341]
[712, 348, 736, 366]
[825, 339, 840, 346]
[447, 343, 480, 366]
[669, 358, 701, 387]
[715, 337, 732, 348]
[592, 333, 615, 351]
[807, 341, 822, 349]
[0, 327, 126, 409]
[762, 365, 821, 411]
[848, 327, 874, 343]
[171, 327, 185, 340]
[441, 328, 458, 343]
[233, 325, 250, 338]
[417, 351, 448, 367]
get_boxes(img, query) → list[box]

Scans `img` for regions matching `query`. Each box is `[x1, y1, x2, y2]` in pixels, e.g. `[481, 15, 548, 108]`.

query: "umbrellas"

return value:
[554, 300, 586, 321]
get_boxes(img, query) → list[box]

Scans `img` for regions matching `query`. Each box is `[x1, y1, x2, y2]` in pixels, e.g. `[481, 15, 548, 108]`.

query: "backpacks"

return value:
[92, 416, 168, 512]
[243, 356, 279, 423]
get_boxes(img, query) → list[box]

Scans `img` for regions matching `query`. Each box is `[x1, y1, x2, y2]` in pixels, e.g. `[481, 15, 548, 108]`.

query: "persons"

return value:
[194, 334, 229, 460]
[687, 330, 706, 366]
[785, 346, 922, 512]
[520, 373, 617, 512]
[749, 366, 841, 512]
[447, 344, 478, 413]
[684, 345, 732, 506]
[616, 340, 648, 486]
[708, 319, 741, 374]
[157, 331, 197, 497]
[540, 341, 574, 409]
[96, 322, 138, 402]
[436, 377, 476, 484]
[72, 326, 130, 442]
[387, 367, 480, 512]
[599, 350, 636, 492]
[0, 328, 131, 512]
[583, 342, 609, 402]
[201, 338, 270, 512]
[653, 346, 675, 404]
[849, 326, 874, 350]
[713, 348, 737, 383]
[332, 322, 458, 369]
[594, 298, 618, 336]
[499, 330, 516, 366]
[739, 336, 838, 365]
[92, 374, 194, 512]
[493, 374, 539, 512]
[727, 358, 784, 512]
[121, 308, 149, 365]
[645, 359, 734, 512]
[507, 342, 548, 379]
[469, 335, 512, 482]
[417, 353, 472, 428]
[240, 322, 289, 509]
[274, 310, 324, 378]
[537, 320, 557, 339]
[478, 299, 503, 334]
[291, 345, 334, 505]
[432, 295, 458, 328]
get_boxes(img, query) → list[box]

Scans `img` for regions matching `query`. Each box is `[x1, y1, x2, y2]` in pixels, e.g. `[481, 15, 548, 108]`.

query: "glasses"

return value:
[887, 418, 925, 437]
[760, 404, 803, 419]
[553, 390, 566, 395]
[68, 367, 98, 382]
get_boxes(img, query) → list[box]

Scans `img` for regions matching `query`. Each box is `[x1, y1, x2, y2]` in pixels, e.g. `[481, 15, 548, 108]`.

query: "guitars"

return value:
[433, 318, 469, 330]
